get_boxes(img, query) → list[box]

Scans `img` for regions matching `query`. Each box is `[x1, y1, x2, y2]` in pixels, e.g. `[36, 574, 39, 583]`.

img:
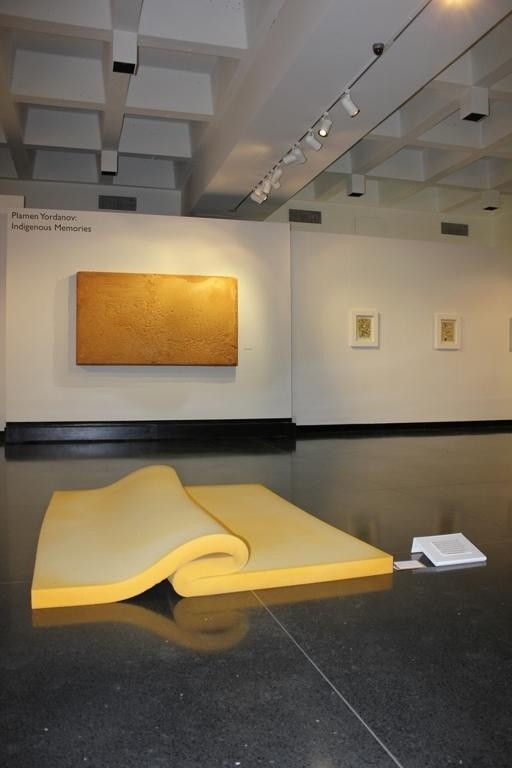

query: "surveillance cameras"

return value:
[373, 43, 384, 55]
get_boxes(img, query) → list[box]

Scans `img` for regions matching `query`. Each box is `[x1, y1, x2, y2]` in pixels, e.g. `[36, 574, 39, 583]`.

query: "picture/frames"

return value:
[433, 312, 463, 350]
[349, 307, 381, 349]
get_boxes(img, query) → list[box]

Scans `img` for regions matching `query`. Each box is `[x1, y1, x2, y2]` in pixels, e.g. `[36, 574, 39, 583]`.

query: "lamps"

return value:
[247, 87, 360, 205]
